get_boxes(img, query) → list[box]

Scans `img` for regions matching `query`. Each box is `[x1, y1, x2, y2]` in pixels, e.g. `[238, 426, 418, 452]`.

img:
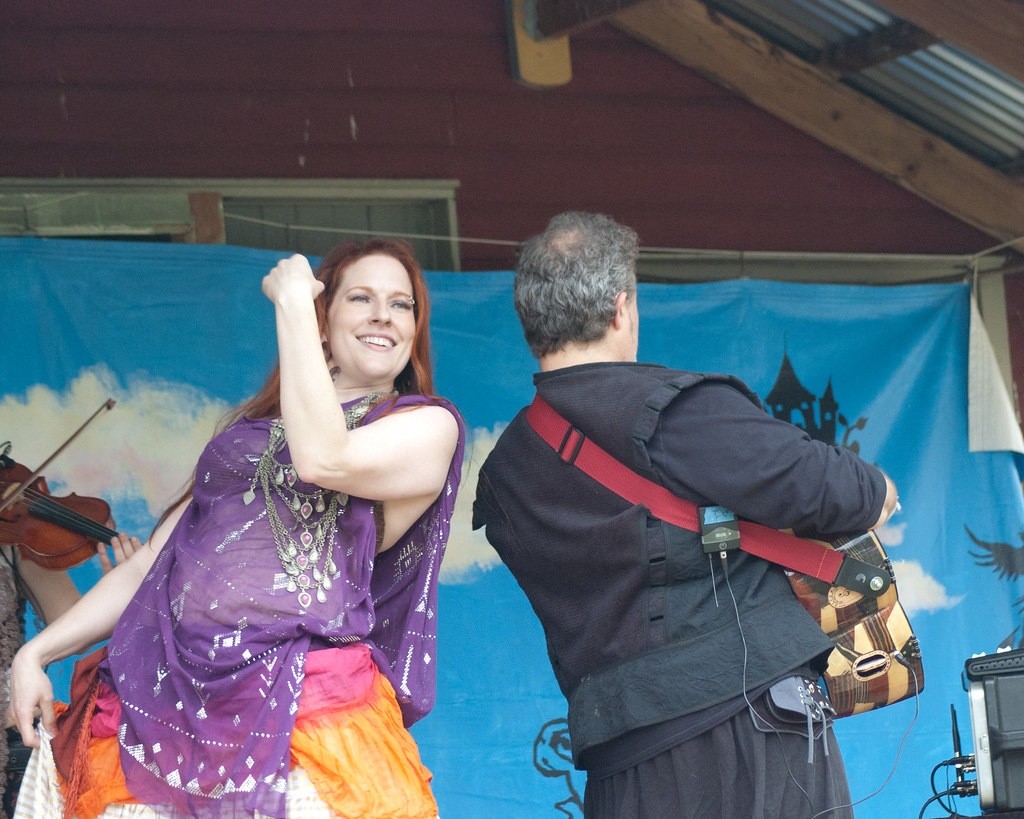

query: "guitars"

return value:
[776, 526, 925, 719]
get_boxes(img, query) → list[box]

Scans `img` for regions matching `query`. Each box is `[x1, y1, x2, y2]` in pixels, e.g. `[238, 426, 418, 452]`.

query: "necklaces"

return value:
[243, 387, 398, 609]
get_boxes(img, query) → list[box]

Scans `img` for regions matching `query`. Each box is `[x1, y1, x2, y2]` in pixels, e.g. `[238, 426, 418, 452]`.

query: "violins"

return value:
[0, 454, 121, 571]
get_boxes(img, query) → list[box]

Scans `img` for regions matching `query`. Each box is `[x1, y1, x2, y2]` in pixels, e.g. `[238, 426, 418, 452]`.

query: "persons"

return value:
[8, 236, 466, 819]
[471, 210, 898, 819]
[0, 530, 142, 819]
